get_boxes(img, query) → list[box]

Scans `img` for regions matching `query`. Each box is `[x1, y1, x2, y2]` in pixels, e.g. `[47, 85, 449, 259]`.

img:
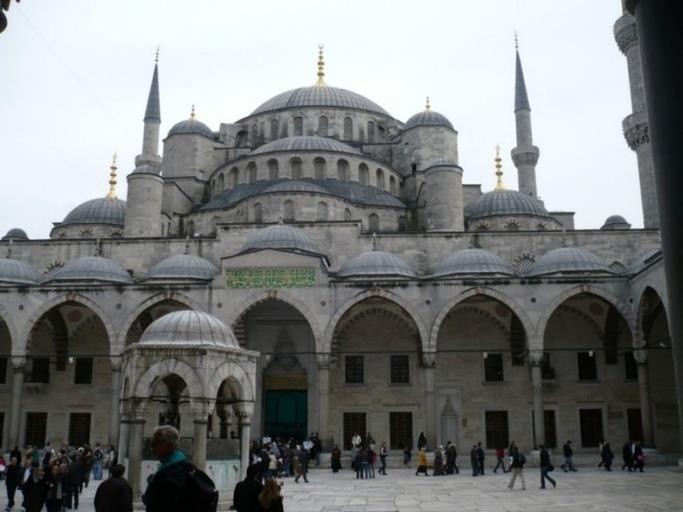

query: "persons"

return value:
[94, 462, 133, 512]
[141, 426, 200, 512]
[494, 439, 526, 491]
[331, 445, 343, 472]
[597, 438, 613, 471]
[249, 431, 322, 484]
[3, 442, 117, 512]
[561, 440, 578, 473]
[233, 465, 285, 512]
[622, 439, 645, 472]
[351, 433, 388, 480]
[404, 432, 459, 476]
[538, 444, 557, 489]
[471, 441, 485, 476]
